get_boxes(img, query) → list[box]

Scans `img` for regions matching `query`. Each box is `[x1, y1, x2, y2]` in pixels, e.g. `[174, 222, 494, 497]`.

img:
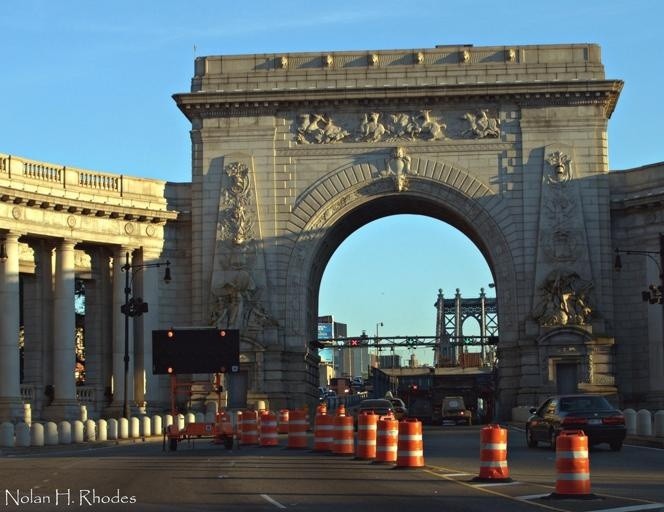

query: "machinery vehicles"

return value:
[150, 325, 242, 452]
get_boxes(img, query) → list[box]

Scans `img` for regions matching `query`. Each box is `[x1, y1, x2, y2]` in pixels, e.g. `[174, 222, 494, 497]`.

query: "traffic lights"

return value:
[351, 339, 358, 346]
[407, 336, 414, 345]
[464, 337, 471, 344]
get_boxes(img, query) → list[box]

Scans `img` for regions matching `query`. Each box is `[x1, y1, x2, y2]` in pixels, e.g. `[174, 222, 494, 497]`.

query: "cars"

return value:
[317, 376, 478, 426]
[525, 394, 627, 452]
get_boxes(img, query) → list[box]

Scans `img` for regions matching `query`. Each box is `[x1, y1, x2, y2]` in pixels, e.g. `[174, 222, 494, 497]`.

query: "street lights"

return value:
[376, 322, 385, 365]
[120, 251, 172, 417]
[612, 232, 664, 324]
